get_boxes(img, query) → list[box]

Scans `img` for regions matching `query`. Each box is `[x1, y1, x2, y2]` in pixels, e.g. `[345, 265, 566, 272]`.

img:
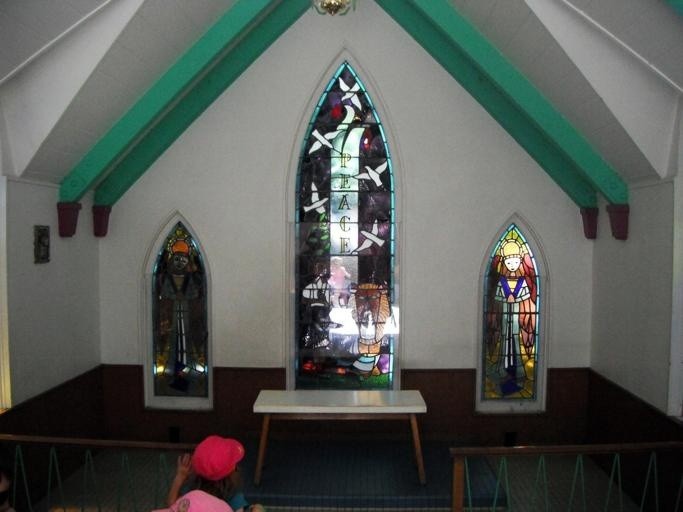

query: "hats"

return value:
[191, 435, 245, 480]
[150, 490, 233, 512]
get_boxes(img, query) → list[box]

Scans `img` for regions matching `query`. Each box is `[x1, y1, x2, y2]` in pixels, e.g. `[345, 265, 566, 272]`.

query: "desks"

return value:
[252, 388, 429, 485]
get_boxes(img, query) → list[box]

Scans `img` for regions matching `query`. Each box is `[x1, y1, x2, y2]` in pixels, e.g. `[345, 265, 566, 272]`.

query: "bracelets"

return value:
[248, 503, 263, 512]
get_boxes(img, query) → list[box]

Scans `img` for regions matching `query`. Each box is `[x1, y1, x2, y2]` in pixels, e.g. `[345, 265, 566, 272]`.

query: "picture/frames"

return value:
[34, 225, 50, 264]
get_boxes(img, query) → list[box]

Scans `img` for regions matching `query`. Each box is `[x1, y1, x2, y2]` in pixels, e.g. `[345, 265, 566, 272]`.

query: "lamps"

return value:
[312, 0, 357, 15]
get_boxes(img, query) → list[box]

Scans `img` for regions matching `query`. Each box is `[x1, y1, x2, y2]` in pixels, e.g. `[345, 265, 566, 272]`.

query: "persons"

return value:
[327, 257, 352, 308]
[166, 436, 264, 512]
